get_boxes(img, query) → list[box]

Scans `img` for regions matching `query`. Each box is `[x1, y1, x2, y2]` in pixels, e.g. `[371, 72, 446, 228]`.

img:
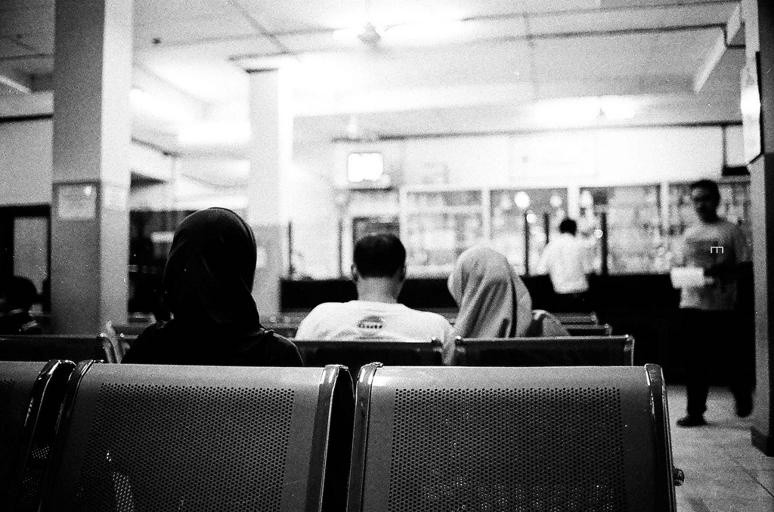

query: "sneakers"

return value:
[677, 414, 707, 426]
[734, 392, 753, 416]
[672, 466, 685, 486]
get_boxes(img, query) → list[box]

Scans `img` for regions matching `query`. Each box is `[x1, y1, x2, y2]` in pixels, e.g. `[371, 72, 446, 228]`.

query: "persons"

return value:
[534, 217, 596, 316]
[116, 208, 306, 366]
[292, 232, 455, 366]
[127, 210, 155, 315]
[671, 178, 755, 428]
[440, 245, 571, 366]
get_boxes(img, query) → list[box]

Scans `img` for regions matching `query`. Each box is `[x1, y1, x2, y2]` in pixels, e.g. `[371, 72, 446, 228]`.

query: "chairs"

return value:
[0, 308, 677, 512]
